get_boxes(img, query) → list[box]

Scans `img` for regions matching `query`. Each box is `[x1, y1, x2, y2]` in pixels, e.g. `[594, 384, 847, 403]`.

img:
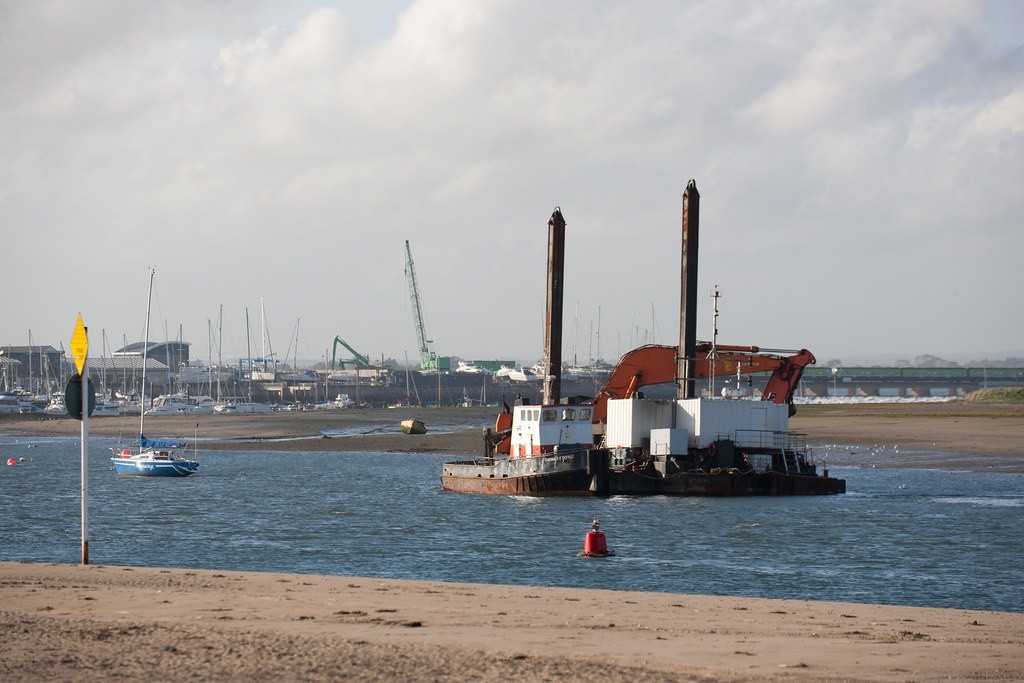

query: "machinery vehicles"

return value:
[404, 238, 459, 372]
[329, 335, 382, 371]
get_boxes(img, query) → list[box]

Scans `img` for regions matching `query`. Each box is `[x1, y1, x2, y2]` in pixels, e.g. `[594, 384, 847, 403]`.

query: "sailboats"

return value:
[0, 296, 350, 417]
[109, 266, 201, 480]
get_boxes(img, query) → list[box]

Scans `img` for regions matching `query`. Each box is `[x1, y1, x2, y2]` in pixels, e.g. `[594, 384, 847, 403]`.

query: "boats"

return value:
[438, 174, 848, 495]
[401, 418, 430, 436]
